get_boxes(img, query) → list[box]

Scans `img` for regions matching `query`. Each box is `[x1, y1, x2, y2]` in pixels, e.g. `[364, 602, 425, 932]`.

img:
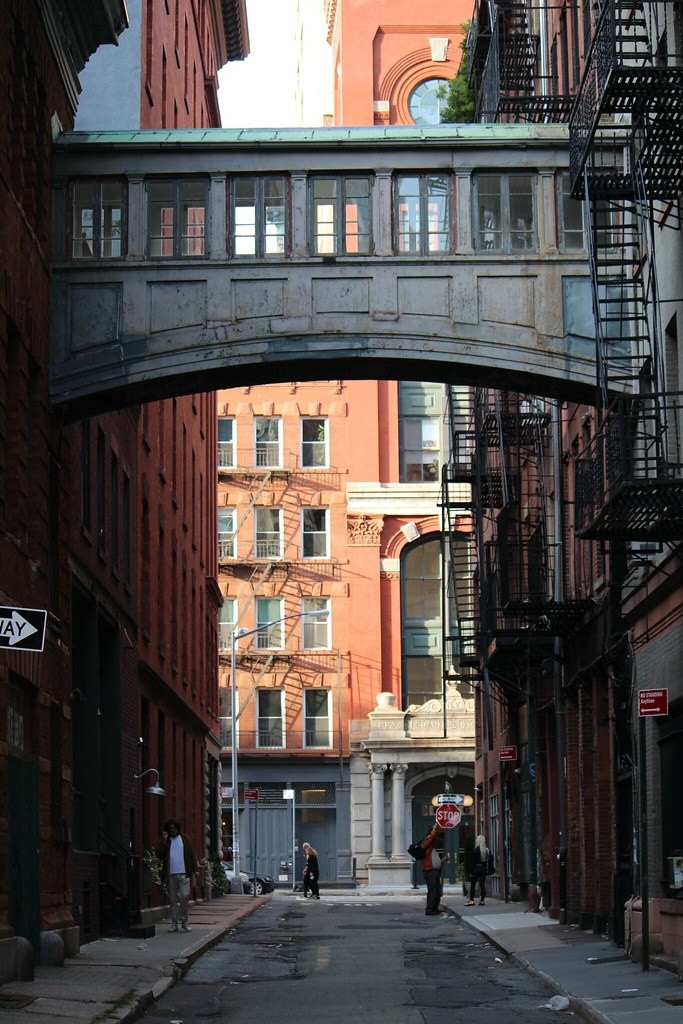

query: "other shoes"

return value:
[181, 922, 192, 932]
[464, 900, 475, 906]
[425, 909, 445, 916]
[167, 924, 178, 933]
[478, 901, 485, 906]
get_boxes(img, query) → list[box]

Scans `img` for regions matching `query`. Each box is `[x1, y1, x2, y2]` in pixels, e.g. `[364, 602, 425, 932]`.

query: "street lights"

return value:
[230, 609, 330, 895]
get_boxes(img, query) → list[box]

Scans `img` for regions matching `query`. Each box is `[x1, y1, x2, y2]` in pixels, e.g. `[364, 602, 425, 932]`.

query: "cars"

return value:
[220, 860, 274, 895]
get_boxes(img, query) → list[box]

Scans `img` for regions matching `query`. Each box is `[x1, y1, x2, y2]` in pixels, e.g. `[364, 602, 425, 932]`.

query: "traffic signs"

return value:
[437, 794, 465, 804]
[0, 606, 48, 652]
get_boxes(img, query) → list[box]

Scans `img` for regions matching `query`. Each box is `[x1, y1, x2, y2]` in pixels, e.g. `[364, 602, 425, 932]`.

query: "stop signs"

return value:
[435, 803, 461, 828]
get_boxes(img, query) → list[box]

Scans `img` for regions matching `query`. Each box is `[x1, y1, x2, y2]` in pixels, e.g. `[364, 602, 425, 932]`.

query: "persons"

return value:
[302, 843, 320, 899]
[464, 835, 490, 906]
[156, 819, 200, 932]
[420, 823, 449, 916]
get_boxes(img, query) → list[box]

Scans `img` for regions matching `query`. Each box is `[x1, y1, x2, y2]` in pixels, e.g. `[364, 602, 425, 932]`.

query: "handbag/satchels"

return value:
[407, 838, 430, 861]
